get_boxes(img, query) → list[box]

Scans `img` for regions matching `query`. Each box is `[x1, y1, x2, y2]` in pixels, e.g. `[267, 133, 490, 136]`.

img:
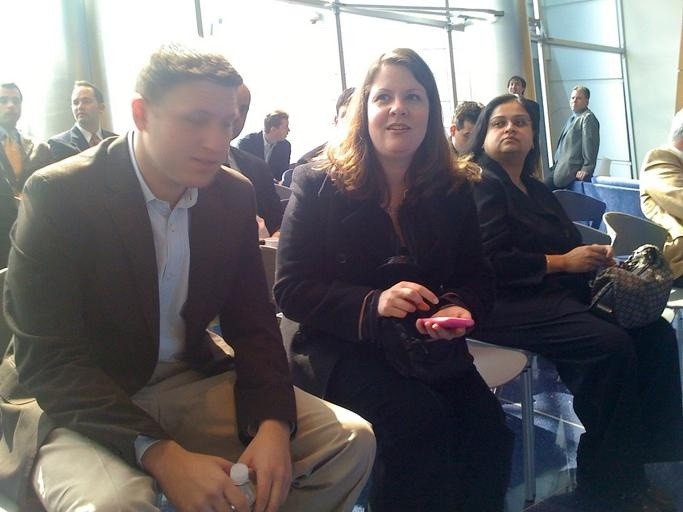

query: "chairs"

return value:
[467, 337, 572, 504]
[602, 212, 682, 368]
[574, 221, 611, 285]
[553, 189, 606, 229]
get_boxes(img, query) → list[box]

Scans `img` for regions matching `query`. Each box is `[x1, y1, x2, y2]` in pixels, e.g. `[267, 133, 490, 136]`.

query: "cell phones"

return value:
[415, 316, 474, 329]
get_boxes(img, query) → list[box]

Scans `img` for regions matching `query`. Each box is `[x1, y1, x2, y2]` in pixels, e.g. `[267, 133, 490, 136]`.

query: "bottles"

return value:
[228, 461, 258, 512]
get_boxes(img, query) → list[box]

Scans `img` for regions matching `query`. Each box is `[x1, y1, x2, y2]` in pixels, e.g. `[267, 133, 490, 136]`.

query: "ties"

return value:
[5, 134, 23, 181]
[91, 134, 99, 146]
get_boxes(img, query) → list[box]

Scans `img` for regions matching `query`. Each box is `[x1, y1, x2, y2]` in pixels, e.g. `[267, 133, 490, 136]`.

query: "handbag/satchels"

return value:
[590, 244, 674, 330]
[359, 256, 470, 384]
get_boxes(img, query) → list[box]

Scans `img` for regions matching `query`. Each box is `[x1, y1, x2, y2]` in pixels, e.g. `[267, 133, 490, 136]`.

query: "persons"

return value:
[508, 76, 542, 174]
[47, 81, 117, 166]
[272, 48, 513, 512]
[639, 108, 682, 288]
[463, 93, 682, 512]
[222, 83, 357, 240]
[0, 81, 51, 274]
[449, 101, 485, 164]
[543, 86, 600, 193]
[0, 44, 376, 512]
